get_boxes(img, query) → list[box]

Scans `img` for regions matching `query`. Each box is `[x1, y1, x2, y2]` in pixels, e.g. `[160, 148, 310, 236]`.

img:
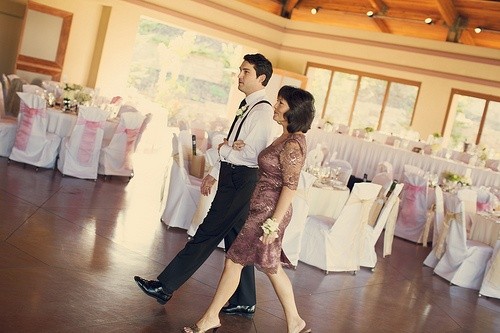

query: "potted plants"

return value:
[441, 170, 471, 189]
[430, 132, 444, 156]
[63, 82, 92, 112]
[475, 148, 487, 168]
[363, 126, 373, 140]
[322, 119, 334, 133]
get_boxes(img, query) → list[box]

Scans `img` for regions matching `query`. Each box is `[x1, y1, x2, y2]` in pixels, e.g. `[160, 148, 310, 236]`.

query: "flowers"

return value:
[261, 218, 280, 239]
[236, 104, 246, 119]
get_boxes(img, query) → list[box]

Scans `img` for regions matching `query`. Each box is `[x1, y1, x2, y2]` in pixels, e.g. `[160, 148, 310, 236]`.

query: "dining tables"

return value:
[299, 185, 350, 220]
[46, 103, 120, 138]
[469, 205, 500, 247]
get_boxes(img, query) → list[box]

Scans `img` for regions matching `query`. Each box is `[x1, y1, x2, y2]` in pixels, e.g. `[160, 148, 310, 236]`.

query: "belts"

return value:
[221, 162, 246, 169]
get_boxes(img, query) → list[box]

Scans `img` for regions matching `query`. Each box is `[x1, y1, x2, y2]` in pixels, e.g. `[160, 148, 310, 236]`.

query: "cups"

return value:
[306, 165, 342, 183]
[468, 156, 478, 165]
[393, 139, 400, 147]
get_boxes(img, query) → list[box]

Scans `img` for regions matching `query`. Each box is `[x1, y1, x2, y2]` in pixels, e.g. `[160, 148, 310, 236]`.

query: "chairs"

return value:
[159, 118, 500, 300]
[0, 74, 153, 181]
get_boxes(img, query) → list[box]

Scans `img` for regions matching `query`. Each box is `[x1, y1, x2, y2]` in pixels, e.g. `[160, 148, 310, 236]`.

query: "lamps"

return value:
[310, 7, 485, 35]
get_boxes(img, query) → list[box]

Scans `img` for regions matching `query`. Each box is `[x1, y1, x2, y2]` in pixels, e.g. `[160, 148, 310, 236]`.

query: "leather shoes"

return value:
[221, 304, 256, 318]
[134, 276, 173, 305]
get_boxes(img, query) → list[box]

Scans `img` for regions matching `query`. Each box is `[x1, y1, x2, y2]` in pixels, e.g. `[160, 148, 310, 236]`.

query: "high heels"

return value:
[300, 328, 312, 333]
[181, 322, 221, 333]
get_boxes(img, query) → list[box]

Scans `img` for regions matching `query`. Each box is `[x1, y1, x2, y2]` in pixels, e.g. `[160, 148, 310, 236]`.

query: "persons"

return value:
[134, 54, 276, 316]
[181, 83, 316, 333]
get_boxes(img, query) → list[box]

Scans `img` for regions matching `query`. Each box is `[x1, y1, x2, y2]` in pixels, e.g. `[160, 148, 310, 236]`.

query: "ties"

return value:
[226, 98, 247, 142]
